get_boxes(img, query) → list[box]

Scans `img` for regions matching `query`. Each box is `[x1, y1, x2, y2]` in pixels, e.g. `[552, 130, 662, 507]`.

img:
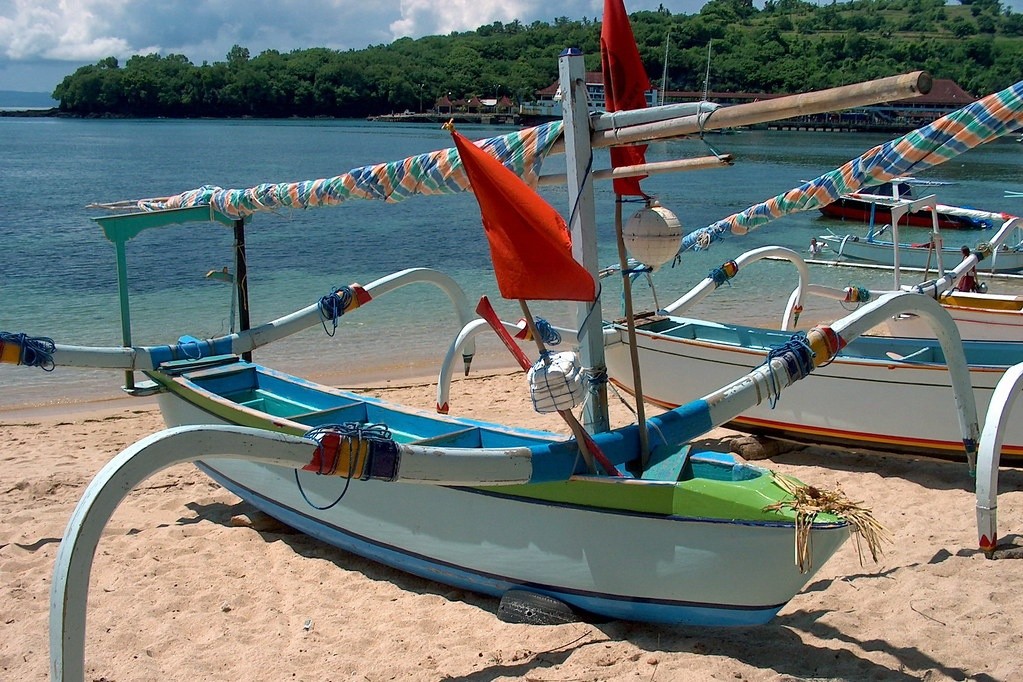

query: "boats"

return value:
[799, 171, 1016, 230]
[436, 80, 1023, 558]
[2, 43, 980, 682]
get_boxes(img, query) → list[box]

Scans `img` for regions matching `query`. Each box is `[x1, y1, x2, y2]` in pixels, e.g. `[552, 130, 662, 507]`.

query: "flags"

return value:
[451, 129, 597, 300]
[602, 2, 654, 196]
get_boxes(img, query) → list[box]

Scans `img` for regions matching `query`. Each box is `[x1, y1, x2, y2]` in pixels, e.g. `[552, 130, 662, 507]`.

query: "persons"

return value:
[808, 236, 828, 252]
[958, 246, 980, 294]
